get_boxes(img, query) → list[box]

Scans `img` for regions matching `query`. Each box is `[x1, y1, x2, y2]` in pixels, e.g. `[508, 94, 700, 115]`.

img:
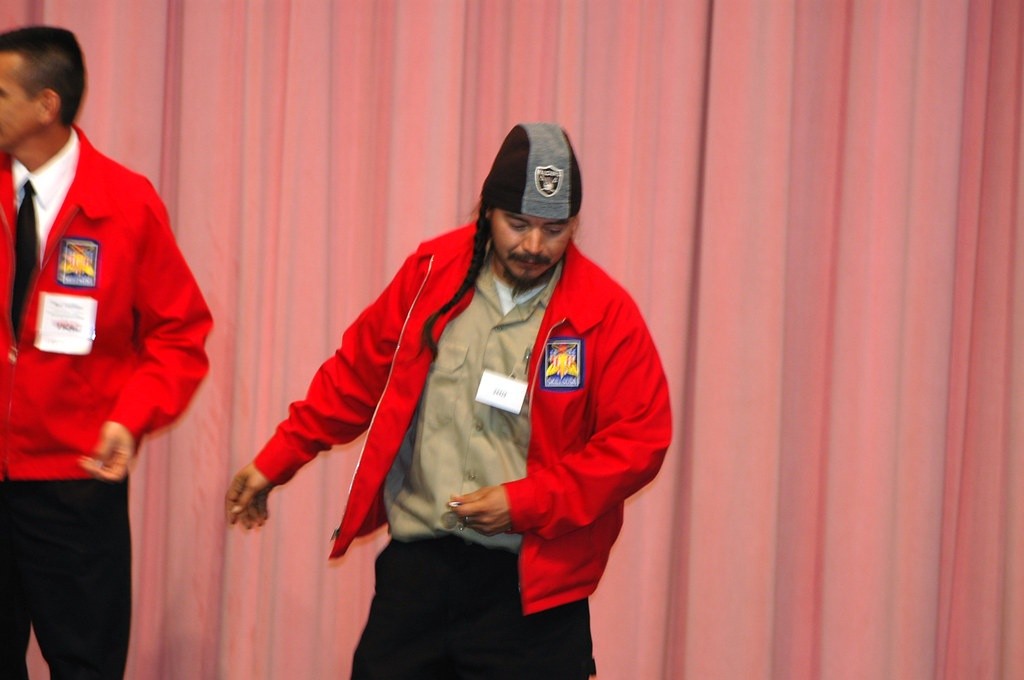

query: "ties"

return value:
[11, 180, 37, 342]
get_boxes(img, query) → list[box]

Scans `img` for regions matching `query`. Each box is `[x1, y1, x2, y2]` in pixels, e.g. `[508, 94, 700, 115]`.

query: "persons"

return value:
[0, 25, 214, 680]
[218, 122, 673, 680]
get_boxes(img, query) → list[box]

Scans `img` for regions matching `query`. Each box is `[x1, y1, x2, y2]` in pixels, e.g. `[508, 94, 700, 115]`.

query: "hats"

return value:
[482, 123, 582, 219]
